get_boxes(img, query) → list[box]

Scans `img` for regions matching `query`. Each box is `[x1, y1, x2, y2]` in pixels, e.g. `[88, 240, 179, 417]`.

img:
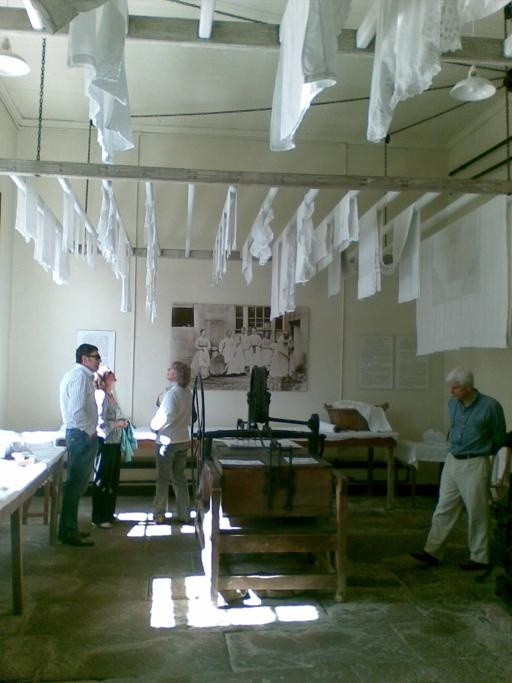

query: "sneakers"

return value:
[90, 515, 191, 529]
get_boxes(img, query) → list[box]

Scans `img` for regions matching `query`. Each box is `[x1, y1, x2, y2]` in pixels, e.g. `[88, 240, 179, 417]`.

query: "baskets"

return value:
[324, 401, 389, 429]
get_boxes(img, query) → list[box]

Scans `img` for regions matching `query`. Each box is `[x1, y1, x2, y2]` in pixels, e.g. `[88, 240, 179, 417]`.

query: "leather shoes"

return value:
[57, 529, 90, 539]
[461, 559, 486, 571]
[410, 549, 439, 568]
[63, 534, 95, 545]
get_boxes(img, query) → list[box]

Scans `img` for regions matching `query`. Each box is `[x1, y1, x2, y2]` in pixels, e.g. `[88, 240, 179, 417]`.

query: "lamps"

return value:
[450, 64, 497, 102]
[0, 34, 30, 77]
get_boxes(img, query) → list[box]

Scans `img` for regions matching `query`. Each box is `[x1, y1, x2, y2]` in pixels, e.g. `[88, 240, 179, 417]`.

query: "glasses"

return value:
[87, 353, 100, 358]
[168, 367, 174, 371]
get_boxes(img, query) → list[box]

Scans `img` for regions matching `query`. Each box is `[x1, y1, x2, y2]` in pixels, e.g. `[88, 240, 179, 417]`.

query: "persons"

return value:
[148, 361, 193, 524]
[191, 326, 296, 379]
[90, 364, 128, 529]
[59, 344, 103, 547]
[409, 369, 507, 570]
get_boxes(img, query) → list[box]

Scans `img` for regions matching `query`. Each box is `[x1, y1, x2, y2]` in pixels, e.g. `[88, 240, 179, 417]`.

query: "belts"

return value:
[455, 453, 481, 458]
